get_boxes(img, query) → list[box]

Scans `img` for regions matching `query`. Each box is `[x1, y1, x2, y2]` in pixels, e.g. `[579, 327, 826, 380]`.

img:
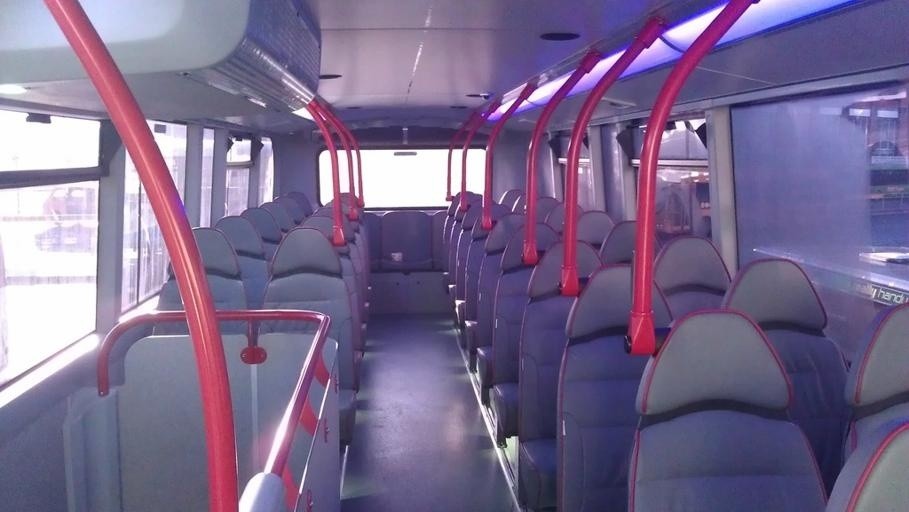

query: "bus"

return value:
[651, 169, 712, 239]
[869, 156, 908, 203]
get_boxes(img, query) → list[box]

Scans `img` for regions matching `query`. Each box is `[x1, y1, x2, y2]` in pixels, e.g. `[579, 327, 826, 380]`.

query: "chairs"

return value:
[157, 227, 249, 336]
[434, 188, 661, 447]
[257, 225, 357, 444]
[629, 309, 826, 512]
[516, 239, 606, 511]
[379, 207, 434, 273]
[720, 256, 852, 498]
[823, 415, 908, 512]
[214, 191, 380, 361]
[651, 236, 733, 320]
[839, 302, 909, 466]
[557, 264, 676, 512]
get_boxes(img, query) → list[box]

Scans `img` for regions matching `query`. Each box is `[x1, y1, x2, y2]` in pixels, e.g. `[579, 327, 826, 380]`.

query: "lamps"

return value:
[482, 1, 857, 123]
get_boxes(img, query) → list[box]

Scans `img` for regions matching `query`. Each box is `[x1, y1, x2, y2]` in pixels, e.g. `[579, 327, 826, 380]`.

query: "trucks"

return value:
[42, 184, 152, 216]
